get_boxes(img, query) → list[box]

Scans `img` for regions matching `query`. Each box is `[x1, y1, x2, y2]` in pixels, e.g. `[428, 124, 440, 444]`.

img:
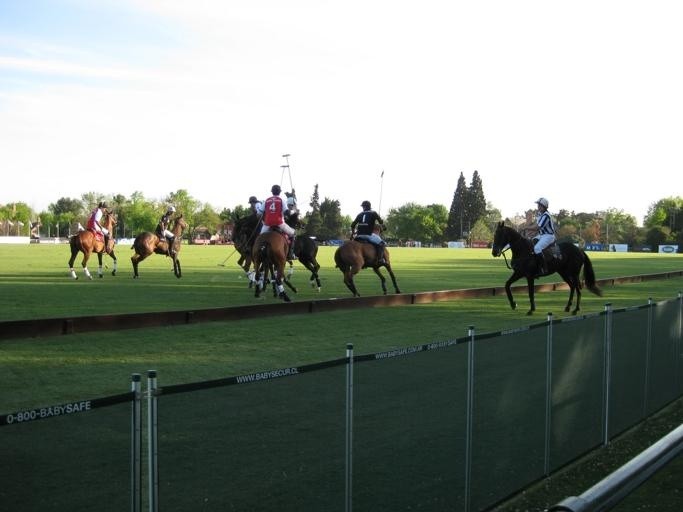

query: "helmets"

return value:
[286, 198, 295, 206]
[248, 196, 256, 204]
[360, 201, 371, 208]
[270, 185, 281, 193]
[167, 205, 175, 213]
[535, 198, 548, 209]
[97, 200, 108, 209]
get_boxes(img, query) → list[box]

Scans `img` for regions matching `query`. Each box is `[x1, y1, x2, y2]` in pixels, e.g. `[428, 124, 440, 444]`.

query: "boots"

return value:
[168, 236, 176, 255]
[104, 233, 110, 253]
[377, 241, 385, 263]
[533, 253, 545, 276]
[287, 237, 297, 260]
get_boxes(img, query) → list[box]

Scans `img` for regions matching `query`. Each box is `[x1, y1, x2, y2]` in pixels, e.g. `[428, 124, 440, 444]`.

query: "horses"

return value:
[232, 210, 321, 302]
[334, 223, 401, 297]
[131, 213, 189, 280]
[492, 221, 603, 316]
[68, 209, 117, 280]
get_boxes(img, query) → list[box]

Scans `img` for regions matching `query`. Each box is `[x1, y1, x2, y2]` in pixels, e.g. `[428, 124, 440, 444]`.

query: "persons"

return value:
[531, 197, 555, 274]
[350, 200, 385, 266]
[247, 196, 261, 215]
[284, 189, 299, 229]
[86, 201, 111, 252]
[257, 185, 296, 260]
[155, 206, 176, 254]
